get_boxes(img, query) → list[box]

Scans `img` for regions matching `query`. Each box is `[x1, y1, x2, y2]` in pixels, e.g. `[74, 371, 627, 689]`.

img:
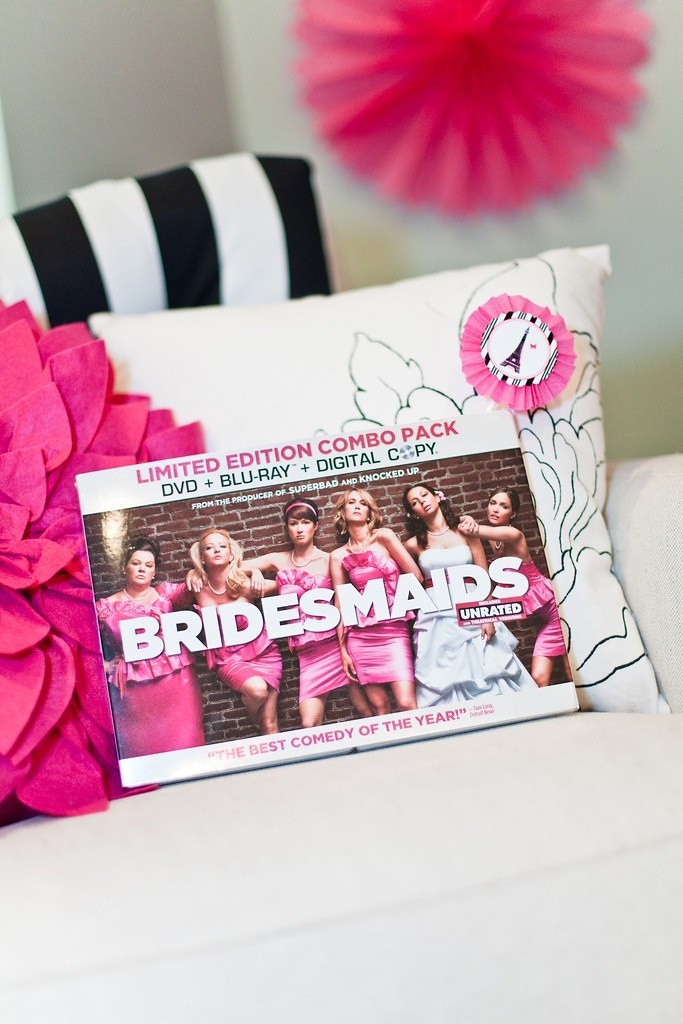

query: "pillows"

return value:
[86, 240, 672, 714]
[0, 153, 334, 334]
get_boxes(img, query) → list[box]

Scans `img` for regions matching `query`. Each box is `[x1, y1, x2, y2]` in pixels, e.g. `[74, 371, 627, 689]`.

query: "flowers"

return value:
[293, 0, 653, 224]
[0, 297, 206, 816]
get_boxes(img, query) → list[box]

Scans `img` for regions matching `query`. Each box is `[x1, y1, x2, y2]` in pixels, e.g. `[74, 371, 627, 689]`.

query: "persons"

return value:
[457, 486, 572, 688]
[328, 487, 423, 715]
[239, 498, 374, 727]
[95, 535, 203, 760]
[187, 530, 282, 734]
[401, 483, 539, 710]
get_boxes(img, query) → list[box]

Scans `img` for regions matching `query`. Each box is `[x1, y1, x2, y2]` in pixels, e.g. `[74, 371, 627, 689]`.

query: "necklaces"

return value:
[349, 533, 370, 554]
[426, 526, 450, 536]
[123, 588, 152, 601]
[208, 580, 226, 595]
[495, 524, 512, 549]
[291, 546, 317, 566]
